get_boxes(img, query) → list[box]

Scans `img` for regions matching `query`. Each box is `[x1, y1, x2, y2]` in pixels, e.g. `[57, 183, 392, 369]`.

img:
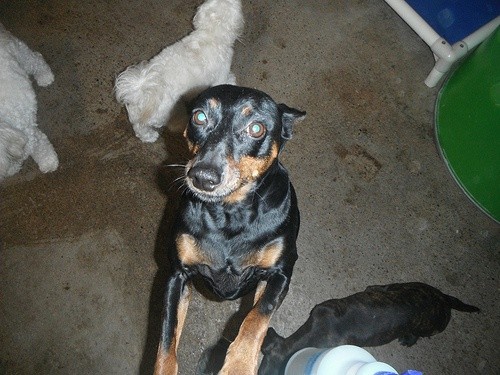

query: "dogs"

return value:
[257, 282, 481, 375]
[114, 0, 247, 144]
[153, 84, 298, 375]
[0, 24, 59, 178]
